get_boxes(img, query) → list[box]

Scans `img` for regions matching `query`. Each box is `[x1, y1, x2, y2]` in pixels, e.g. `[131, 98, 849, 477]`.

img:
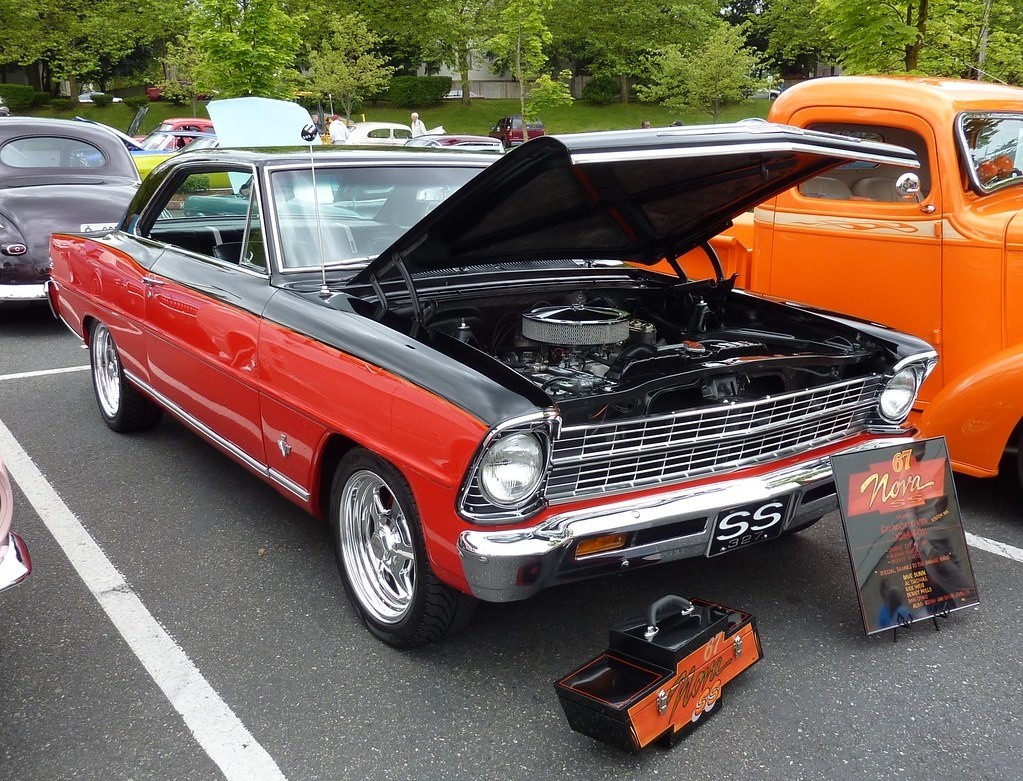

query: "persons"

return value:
[641, 120, 652, 129]
[669, 120, 683, 127]
[328, 114, 349, 145]
[410, 112, 428, 138]
[312, 112, 319, 128]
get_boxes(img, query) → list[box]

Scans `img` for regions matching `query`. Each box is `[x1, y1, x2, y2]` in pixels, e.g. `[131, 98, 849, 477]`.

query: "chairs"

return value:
[211, 240, 268, 269]
[853, 176, 924, 202]
[800, 176, 855, 202]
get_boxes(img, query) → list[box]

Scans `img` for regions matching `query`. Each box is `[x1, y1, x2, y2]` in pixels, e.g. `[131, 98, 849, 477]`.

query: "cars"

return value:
[77, 92, 123, 104]
[293, 91, 323, 103]
[76, 104, 505, 222]
[0, 118, 177, 300]
[742, 80, 780, 101]
[445, 89, 486, 99]
[46, 122, 938, 652]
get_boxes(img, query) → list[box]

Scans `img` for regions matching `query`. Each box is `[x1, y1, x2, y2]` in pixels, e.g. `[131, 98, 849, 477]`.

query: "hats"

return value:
[670, 121, 682, 127]
[332, 115, 338, 119]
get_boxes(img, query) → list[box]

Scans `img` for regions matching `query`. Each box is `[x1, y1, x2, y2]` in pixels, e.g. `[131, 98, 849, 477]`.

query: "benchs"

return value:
[151, 226, 401, 263]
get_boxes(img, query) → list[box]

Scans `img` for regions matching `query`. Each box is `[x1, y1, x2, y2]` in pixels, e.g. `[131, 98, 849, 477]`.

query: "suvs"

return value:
[489, 118, 544, 146]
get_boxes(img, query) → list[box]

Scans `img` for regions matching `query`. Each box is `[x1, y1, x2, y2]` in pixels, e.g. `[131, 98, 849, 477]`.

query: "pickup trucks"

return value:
[148, 81, 220, 99]
[628, 74, 1023, 481]
